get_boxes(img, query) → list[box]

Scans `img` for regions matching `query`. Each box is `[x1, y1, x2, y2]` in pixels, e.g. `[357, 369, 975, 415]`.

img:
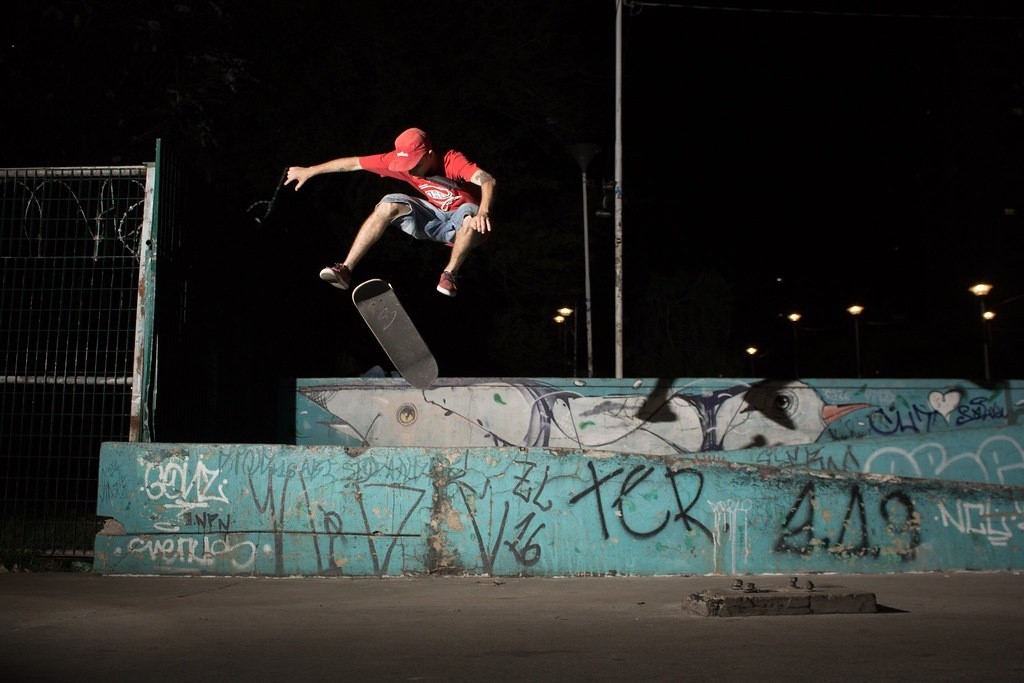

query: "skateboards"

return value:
[352, 277, 439, 388]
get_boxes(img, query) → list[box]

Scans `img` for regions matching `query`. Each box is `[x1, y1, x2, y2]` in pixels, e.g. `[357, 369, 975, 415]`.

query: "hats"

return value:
[388, 128, 429, 171]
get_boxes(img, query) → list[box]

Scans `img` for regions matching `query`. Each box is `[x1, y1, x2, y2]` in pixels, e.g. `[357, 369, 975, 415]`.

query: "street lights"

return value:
[554, 304, 578, 377]
[847, 304, 864, 378]
[746, 346, 758, 377]
[968, 283, 994, 382]
[788, 312, 802, 379]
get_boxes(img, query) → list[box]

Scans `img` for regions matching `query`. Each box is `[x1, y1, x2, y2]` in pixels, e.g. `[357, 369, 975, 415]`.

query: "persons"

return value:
[284, 128, 495, 297]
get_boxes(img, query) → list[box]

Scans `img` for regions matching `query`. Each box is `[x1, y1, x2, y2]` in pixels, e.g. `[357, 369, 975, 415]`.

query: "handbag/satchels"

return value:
[458, 181, 502, 201]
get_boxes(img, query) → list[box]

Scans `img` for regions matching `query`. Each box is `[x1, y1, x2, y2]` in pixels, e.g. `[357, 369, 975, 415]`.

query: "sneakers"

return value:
[320, 263, 351, 289]
[436, 271, 457, 297]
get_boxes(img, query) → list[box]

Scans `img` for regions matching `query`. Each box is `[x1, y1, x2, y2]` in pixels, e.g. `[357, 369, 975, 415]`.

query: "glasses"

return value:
[409, 156, 425, 173]
